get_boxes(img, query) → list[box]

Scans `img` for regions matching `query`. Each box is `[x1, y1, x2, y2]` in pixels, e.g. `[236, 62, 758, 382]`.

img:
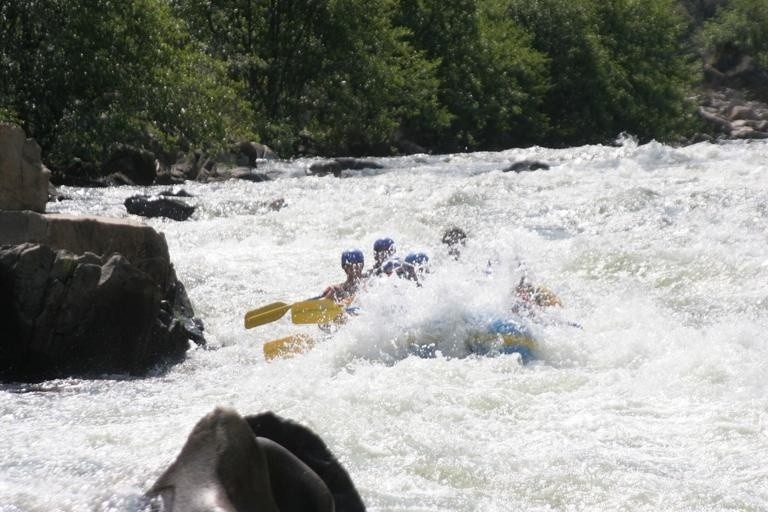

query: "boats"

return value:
[471, 284, 559, 364]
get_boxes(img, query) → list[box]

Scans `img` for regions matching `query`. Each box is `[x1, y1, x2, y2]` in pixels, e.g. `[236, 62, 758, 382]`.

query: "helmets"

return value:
[374, 238, 396, 254]
[405, 252, 429, 267]
[341, 249, 364, 268]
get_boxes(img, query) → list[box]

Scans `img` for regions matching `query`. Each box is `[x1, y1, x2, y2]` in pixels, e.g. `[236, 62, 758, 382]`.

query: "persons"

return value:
[313, 220, 571, 330]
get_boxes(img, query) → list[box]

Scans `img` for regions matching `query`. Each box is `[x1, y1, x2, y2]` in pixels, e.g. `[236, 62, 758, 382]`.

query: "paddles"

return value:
[291, 300, 360, 324]
[264, 333, 335, 362]
[245, 294, 325, 329]
